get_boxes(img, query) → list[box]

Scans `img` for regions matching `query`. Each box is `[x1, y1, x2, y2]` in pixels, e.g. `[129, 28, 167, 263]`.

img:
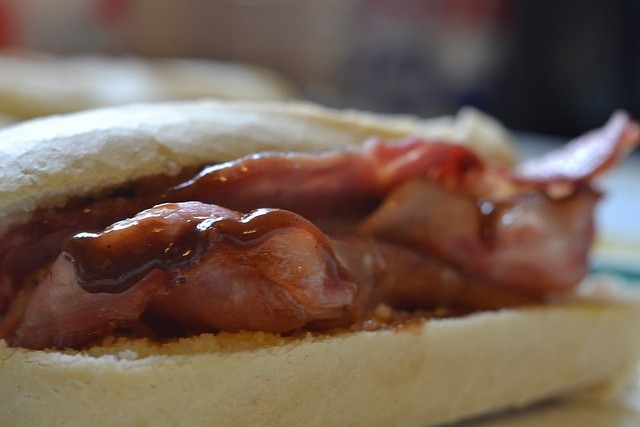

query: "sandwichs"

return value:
[1, 100, 639, 427]
